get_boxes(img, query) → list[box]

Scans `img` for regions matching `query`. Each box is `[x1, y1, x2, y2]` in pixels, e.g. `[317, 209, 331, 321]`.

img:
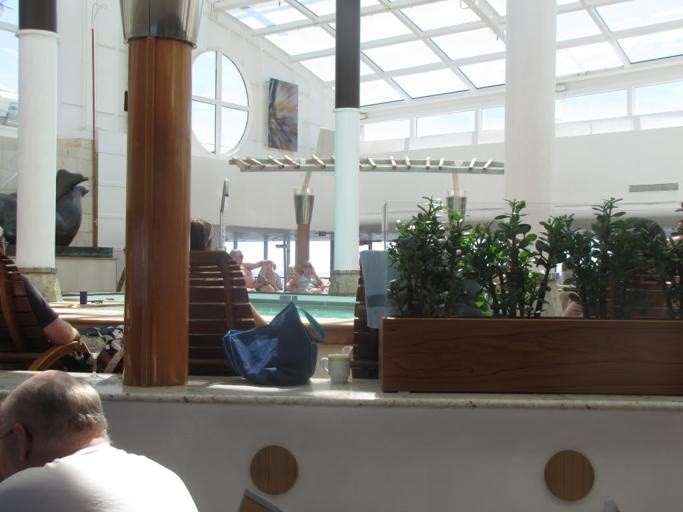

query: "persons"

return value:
[229, 248, 324, 293]
[190, 218, 268, 328]
[0, 219, 88, 371]
[0, 370, 200, 512]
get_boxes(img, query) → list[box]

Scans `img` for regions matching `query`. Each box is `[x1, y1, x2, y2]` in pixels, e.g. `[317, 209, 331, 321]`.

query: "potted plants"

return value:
[378, 195, 683, 396]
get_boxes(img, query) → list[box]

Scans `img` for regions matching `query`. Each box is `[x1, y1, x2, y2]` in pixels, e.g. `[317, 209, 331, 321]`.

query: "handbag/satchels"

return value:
[222, 301, 325, 386]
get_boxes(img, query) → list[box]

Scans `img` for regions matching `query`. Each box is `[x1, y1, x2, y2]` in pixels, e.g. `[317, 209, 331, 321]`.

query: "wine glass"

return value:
[82, 337, 108, 380]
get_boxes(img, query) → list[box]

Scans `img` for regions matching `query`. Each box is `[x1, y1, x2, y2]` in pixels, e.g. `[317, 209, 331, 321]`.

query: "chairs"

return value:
[0, 255, 88, 373]
[289, 264, 316, 278]
[188, 249, 256, 375]
[351, 249, 394, 380]
[605, 270, 680, 321]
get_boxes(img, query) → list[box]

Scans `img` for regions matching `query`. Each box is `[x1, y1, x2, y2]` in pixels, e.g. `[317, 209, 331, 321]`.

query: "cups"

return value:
[320, 354, 351, 384]
[80, 290, 87, 305]
